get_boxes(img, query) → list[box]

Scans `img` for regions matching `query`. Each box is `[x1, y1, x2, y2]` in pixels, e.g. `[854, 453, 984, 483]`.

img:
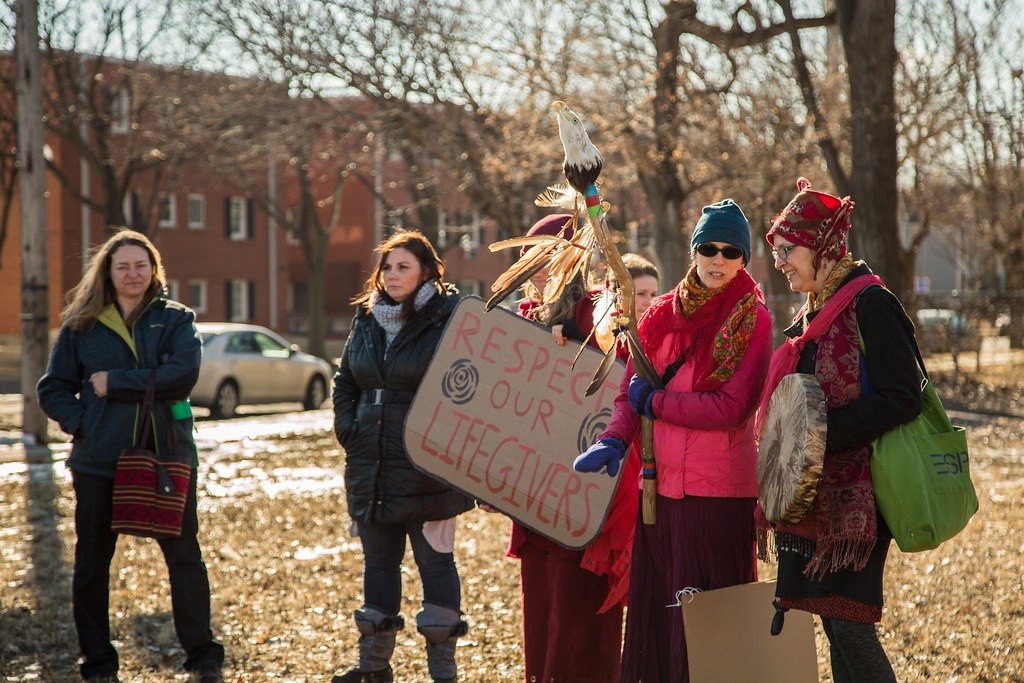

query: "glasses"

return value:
[696, 242, 743, 259]
[771, 244, 799, 260]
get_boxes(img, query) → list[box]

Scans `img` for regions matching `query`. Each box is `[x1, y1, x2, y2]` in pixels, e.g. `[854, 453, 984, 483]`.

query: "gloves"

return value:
[627, 373, 664, 421]
[572, 437, 626, 478]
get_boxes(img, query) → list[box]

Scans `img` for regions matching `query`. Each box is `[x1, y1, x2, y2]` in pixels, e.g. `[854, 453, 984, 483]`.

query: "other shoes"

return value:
[188, 655, 224, 683]
[84, 667, 121, 683]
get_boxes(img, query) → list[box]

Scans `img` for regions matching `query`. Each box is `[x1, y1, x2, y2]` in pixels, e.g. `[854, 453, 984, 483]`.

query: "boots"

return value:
[414, 599, 468, 683]
[331, 603, 405, 683]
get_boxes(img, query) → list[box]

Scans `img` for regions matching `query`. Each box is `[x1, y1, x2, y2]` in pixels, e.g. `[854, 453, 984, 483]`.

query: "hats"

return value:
[520, 214, 575, 263]
[766, 176, 856, 263]
[690, 198, 751, 268]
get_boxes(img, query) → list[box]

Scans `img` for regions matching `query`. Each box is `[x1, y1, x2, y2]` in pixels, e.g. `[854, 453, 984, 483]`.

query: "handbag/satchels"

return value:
[109, 401, 192, 539]
[853, 282, 979, 553]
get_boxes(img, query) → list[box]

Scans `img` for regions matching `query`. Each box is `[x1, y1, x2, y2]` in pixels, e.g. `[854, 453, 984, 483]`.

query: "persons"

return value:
[755, 176, 923, 683]
[331, 225, 478, 683]
[35, 224, 225, 683]
[573, 199, 774, 683]
[479, 213, 659, 683]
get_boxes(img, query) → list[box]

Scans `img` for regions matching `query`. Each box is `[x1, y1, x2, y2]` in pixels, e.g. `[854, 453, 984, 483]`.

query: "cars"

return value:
[188, 321, 336, 422]
